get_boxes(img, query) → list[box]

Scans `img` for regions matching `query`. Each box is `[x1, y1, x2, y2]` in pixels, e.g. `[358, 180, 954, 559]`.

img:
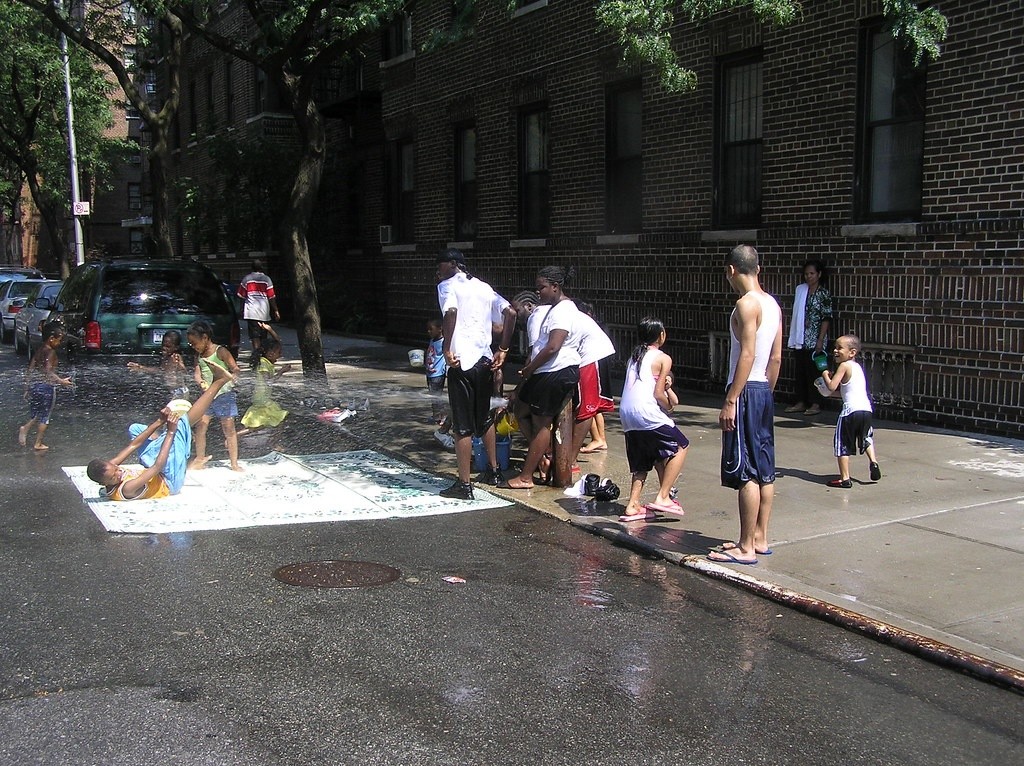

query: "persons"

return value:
[707, 244, 782, 564]
[424, 248, 616, 499]
[237, 258, 280, 348]
[822, 334, 882, 488]
[87, 320, 292, 501]
[18, 322, 74, 450]
[784, 260, 833, 415]
[619, 316, 690, 521]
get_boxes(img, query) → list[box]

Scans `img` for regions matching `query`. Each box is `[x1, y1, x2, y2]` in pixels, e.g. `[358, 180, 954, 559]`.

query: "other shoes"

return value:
[869, 462, 881, 480]
[827, 479, 852, 488]
[423, 413, 448, 425]
[293, 395, 371, 425]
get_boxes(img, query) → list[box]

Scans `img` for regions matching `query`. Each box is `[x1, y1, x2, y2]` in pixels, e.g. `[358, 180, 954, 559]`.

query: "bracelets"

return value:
[498, 344, 509, 352]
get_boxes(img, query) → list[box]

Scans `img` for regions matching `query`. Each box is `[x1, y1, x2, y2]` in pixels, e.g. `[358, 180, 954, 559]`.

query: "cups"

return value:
[814, 377, 833, 397]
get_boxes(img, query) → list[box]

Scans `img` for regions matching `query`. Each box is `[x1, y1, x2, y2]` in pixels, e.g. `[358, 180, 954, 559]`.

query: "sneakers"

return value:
[581, 473, 620, 501]
[478, 469, 500, 485]
[434, 428, 455, 447]
[439, 481, 475, 499]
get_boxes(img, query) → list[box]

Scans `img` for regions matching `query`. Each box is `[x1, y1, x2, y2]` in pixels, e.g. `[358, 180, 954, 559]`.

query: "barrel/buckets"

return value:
[408, 349, 424, 366]
[496, 414, 519, 435]
[812, 350, 828, 371]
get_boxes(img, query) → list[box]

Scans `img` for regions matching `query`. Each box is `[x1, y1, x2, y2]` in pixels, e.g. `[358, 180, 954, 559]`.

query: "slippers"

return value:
[534, 461, 553, 485]
[784, 406, 805, 412]
[707, 551, 759, 565]
[579, 443, 608, 453]
[803, 410, 821, 415]
[715, 541, 772, 555]
[648, 499, 685, 515]
[620, 505, 656, 521]
[497, 480, 531, 489]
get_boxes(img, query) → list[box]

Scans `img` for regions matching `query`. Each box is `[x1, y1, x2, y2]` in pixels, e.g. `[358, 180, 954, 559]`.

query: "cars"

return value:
[41, 260, 247, 374]
[0, 265, 64, 356]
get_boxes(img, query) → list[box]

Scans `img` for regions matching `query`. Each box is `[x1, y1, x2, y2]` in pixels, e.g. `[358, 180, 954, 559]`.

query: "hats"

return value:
[435, 247, 464, 262]
